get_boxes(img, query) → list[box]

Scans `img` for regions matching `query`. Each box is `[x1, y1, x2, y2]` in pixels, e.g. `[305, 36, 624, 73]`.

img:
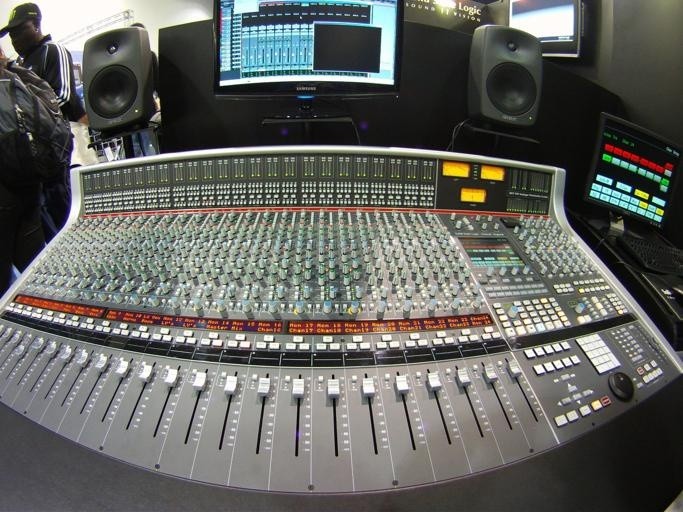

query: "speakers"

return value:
[464, 24, 543, 142]
[83, 27, 154, 148]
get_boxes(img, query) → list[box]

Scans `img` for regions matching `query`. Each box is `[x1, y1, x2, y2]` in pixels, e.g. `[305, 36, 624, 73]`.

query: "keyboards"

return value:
[616, 236, 683, 276]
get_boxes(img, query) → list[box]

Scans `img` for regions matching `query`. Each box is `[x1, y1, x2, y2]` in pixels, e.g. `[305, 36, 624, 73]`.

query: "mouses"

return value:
[670, 283, 683, 305]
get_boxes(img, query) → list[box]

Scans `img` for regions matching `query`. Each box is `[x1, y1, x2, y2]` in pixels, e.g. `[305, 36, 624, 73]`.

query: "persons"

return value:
[0, 50, 72, 293]
[0, 3, 85, 232]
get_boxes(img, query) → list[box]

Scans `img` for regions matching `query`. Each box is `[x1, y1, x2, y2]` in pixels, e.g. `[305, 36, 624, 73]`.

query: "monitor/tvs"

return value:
[213, 0, 404, 123]
[509, 0, 583, 59]
[582, 111, 683, 238]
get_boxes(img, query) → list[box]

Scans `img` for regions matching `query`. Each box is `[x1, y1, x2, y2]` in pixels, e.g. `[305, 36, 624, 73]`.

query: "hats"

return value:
[0, 4, 41, 38]
